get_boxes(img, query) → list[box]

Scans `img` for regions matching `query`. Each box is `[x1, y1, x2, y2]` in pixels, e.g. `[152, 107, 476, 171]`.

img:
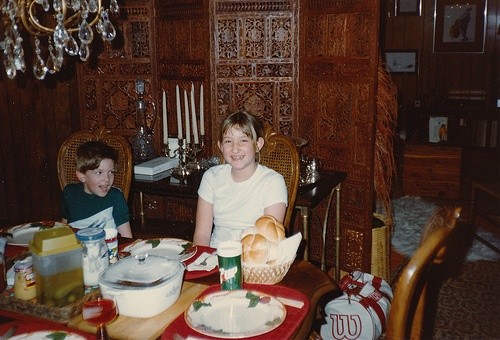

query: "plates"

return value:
[183, 289, 288, 339]
[117, 237, 199, 262]
[6, 221, 68, 250]
[5, 330, 88, 340]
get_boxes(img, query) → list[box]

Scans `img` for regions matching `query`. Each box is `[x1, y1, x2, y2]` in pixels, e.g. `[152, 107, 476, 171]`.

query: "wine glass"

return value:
[82, 291, 119, 339]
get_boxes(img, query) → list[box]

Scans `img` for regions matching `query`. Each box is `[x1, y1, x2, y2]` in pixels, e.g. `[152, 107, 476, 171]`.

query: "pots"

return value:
[95, 253, 185, 319]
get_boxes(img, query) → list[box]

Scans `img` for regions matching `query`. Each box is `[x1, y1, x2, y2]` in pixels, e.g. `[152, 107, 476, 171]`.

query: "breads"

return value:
[241, 214, 286, 263]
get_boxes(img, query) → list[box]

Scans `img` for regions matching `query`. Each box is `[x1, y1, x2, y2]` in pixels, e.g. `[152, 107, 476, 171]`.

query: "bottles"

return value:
[13, 260, 37, 305]
[104, 228, 118, 264]
[77, 227, 110, 285]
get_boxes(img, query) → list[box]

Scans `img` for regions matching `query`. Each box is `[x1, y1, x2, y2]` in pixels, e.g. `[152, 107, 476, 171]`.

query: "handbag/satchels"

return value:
[320, 270, 394, 340]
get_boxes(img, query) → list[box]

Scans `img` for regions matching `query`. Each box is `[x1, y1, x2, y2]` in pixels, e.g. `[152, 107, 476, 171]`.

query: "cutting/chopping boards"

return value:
[67, 280, 213, 340]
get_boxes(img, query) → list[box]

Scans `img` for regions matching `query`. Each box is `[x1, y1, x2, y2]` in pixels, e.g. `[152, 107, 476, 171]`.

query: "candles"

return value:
[189, 83, 199, 145]
[174, 83, 184, 139]
[160, 86, 169, 146]
[200, 84, 205, 136]
[184, 90, 191, 145]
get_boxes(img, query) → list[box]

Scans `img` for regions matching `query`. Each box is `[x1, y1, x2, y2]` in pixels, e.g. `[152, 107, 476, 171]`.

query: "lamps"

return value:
[1, 1, 121, 82]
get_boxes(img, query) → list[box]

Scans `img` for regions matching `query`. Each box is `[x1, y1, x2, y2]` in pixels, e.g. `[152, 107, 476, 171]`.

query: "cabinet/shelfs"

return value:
[393, 144, 461, 205]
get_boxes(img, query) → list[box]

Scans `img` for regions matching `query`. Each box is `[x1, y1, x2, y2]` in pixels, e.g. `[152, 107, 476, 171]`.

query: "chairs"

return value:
[313, 205, 463, 339]
[215, 123, 302, 252]
[56, 126, 134, 217]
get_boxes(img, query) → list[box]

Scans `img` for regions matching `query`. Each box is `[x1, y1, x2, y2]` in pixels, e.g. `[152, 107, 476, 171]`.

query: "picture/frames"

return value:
[431, 1, 488, 55]
[381, 48, 421, 76]
[393, 1, 422, 16]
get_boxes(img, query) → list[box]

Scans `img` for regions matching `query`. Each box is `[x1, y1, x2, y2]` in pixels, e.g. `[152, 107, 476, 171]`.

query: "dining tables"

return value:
[0, 215, 311, 340]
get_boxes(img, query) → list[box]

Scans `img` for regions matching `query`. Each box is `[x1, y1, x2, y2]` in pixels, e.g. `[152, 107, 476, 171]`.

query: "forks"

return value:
[193, 250, 218, 267]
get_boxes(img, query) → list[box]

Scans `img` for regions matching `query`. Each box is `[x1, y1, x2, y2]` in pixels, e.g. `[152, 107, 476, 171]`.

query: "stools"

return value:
[467, 180, 500, 258]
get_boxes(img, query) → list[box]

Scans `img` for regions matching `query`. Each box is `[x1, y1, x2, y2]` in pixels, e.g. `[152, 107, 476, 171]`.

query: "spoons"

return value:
[118, 238, 143, 257]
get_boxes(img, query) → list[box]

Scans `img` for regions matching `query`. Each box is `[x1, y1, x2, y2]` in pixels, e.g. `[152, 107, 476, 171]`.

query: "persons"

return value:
[58, 141, 136, 241]
[439, 123, 447, 142]
[193, 111, 289, 250]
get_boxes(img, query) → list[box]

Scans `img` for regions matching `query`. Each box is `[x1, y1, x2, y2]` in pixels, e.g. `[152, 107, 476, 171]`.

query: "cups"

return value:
[215, 240, 244, 292]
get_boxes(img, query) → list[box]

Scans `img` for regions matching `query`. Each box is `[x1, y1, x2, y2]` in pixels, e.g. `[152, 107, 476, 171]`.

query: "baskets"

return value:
[243, 254, 296, 285]
